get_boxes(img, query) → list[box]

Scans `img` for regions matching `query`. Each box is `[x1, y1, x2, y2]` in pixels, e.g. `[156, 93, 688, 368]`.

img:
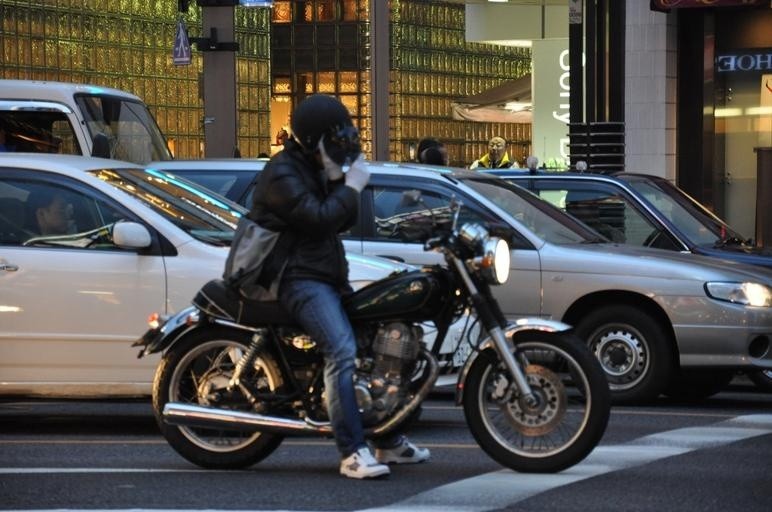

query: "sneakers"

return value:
[339, 449, 390, 480]
[373, 439, 430, 463]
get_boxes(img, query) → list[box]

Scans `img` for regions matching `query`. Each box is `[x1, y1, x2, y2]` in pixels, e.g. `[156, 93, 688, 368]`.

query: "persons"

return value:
[222, 93, 431, 480]
[24, 187, 73, 238]
[565, 191, 627, 243]
[470, 136, 520, 170]
[393, 188, 425, 215]
[417, 135, 449, 168]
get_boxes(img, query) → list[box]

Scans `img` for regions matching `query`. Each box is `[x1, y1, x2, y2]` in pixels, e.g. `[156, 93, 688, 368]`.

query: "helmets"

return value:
[290, 93, 360, 164]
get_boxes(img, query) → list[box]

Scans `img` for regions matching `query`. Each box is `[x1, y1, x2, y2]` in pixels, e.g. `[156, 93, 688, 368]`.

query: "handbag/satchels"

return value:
[225, 217, 292, 302]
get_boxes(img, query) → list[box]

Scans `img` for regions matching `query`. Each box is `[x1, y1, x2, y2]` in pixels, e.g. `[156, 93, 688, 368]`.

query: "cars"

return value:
[0, 154, 481, 403]
[145, 159, 772, 406]
[467, 164, 772, 274]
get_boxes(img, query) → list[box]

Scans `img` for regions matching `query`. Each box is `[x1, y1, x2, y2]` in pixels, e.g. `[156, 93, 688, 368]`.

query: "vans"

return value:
[0, 78, 176, 168]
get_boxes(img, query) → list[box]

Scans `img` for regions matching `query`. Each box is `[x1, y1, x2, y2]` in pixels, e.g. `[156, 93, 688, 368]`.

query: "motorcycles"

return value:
[132, 189, 611, 472]
